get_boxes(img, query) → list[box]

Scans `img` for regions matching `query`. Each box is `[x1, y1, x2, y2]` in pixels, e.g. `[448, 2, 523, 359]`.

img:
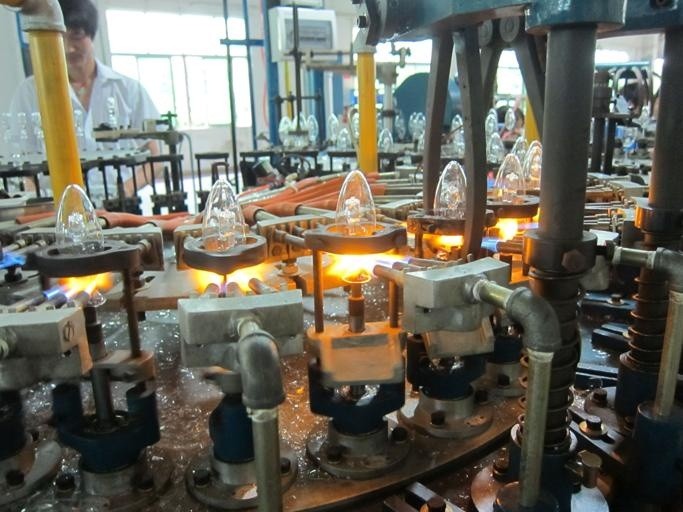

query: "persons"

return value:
[0, 0, 165, 208]
[495, 105, 525, 141]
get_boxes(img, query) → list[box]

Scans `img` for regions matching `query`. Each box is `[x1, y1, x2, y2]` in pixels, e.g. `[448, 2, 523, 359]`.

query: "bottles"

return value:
[0, 112, 45, 162]
[74, 110, 85, 154]
